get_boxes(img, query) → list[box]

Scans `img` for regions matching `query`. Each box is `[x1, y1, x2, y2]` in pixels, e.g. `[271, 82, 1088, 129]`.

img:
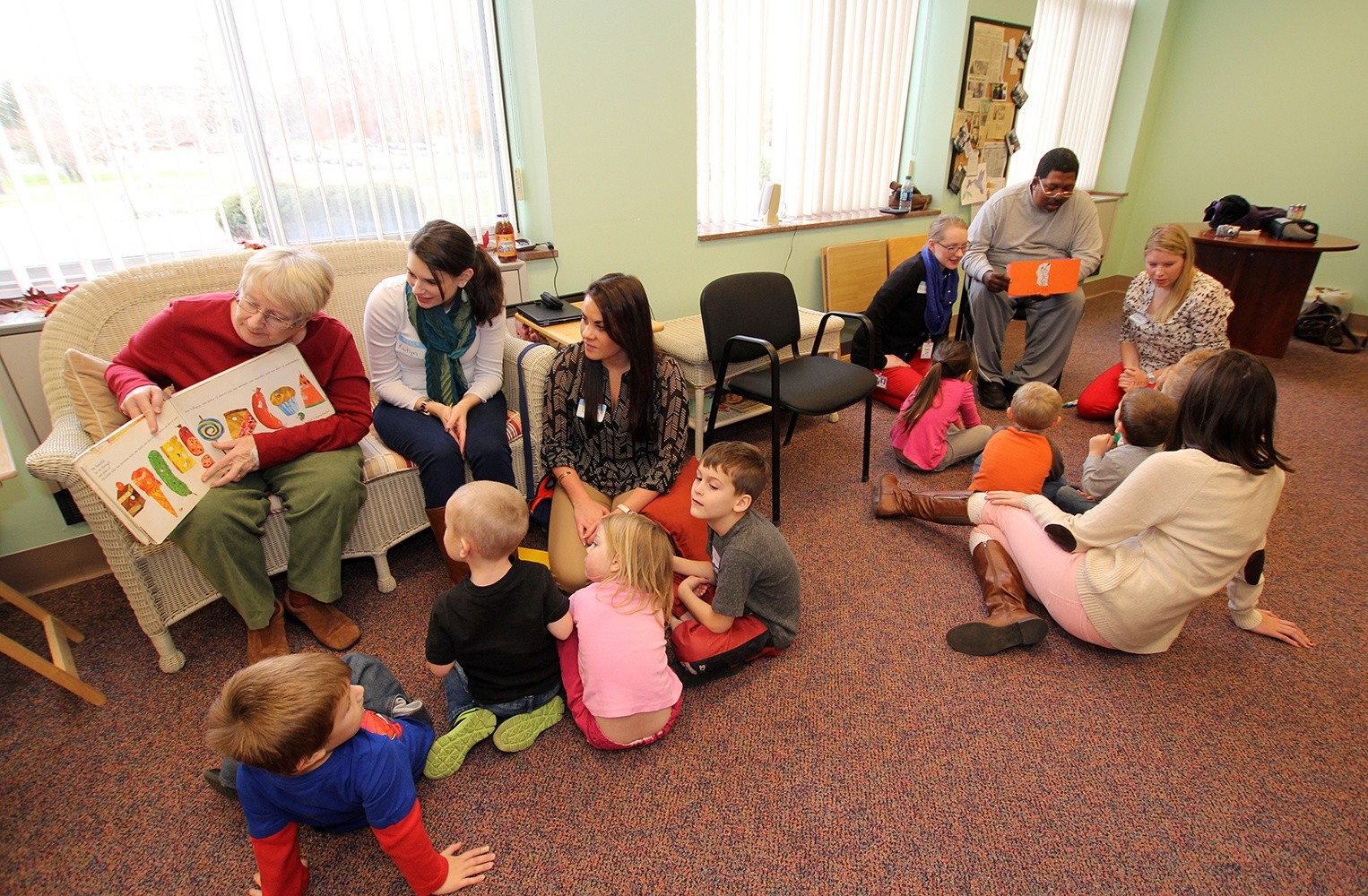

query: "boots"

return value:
[426, 506, 467, 588]
[873, 471, 977, 527]
[945, 539, 1046, 655]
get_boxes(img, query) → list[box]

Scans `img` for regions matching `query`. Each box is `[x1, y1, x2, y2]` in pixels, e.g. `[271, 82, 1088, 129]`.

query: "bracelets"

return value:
[557, 471, 578, 488]
[419, 399, 436, 416]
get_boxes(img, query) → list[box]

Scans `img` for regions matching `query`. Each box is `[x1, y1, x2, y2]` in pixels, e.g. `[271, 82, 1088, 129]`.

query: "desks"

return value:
[1152, 217, 1361, 360]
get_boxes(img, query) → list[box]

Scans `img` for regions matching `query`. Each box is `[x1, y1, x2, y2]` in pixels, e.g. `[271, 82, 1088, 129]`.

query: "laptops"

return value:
[516, 296, 584, 328]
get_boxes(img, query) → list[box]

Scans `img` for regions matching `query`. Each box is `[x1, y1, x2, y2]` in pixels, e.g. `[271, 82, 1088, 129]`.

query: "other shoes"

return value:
[245, 597, 290, 666]
[285, 589, 361, 651]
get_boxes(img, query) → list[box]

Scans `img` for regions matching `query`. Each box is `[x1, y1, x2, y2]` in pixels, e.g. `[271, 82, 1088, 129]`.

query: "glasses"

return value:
[935, 239, 972, 253]
[232, 287, 307, 331]
[1036, 175, 1075, 198]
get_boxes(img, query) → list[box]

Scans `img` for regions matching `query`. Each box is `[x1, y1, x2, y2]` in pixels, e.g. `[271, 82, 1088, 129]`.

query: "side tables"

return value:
[0, 399, 109, 709]
[512, 290, 665, 352]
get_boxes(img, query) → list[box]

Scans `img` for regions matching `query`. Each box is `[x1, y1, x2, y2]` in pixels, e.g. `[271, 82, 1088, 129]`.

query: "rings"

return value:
[236, 474, 244, 479]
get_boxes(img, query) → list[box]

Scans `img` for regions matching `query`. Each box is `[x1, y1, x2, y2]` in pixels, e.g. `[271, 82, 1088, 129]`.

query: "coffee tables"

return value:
[651, 305, 846, 465]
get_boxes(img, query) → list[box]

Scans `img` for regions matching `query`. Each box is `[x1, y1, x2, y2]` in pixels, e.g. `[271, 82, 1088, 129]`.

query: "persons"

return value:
[419, 481, 575, 781]
[101, 245, 373, 666]
[202, 652, 496, 896]
[670, 441, 801, 682]
[1155, 347, 1225, 409]
[872, 349, 1316, 659]
[851, 215, 971, 414]
[890, 339, 995, 473]
[1055, 385, 1177, 515]
[1076, 222, 1235, 420]
[959, 146, 1104, 409]
[540, 273, 694, 593]
[967, 380, 1068, 502]
[554, 511, 684, 751]
[361, 220, 518, 584]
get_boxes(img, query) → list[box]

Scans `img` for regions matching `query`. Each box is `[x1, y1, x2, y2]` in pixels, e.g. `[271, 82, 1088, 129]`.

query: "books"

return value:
[71, 343, 336, 546]
[722, 401, 766, 412]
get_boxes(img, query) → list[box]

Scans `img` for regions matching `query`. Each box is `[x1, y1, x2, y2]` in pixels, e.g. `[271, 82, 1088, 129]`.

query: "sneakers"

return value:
[1003, 379, 1021, 403]
[203, 769, 238, 801]
[421, 705, 496, 780]
[976, 373, 1005, 409]
[494, 695, 564, 753]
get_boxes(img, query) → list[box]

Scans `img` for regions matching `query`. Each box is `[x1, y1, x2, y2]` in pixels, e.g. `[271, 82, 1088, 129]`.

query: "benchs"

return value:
[27, 238, 560, 676]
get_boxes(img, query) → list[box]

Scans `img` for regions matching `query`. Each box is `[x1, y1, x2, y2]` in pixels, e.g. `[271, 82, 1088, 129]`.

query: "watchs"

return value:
[1147, 371, 1157, 385]
[616, 504, 638, 516]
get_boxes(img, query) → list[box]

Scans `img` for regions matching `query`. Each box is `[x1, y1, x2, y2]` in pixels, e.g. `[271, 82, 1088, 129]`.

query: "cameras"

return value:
[1216, 224, 1241, 239]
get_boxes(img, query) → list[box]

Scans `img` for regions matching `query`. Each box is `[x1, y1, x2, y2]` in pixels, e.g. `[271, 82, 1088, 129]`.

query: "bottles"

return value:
[899, 176, 914, 213]
[496, 213, 517, 263]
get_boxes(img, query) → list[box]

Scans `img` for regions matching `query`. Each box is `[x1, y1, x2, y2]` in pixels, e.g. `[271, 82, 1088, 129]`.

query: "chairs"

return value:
[699, 272, 876, 528]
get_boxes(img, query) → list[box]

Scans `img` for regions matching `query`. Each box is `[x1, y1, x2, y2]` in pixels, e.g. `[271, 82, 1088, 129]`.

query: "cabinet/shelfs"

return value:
[490, 254, 531, 340]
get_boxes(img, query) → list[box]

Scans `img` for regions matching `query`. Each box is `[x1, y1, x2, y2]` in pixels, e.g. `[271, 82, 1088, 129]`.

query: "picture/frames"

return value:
[947, 15, 1032, 193]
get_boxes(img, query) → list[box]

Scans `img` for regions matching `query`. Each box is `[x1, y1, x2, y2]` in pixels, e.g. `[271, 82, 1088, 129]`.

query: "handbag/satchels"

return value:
[1295, 296, 1368, 354]
[1298, 287, 1352, 323]
